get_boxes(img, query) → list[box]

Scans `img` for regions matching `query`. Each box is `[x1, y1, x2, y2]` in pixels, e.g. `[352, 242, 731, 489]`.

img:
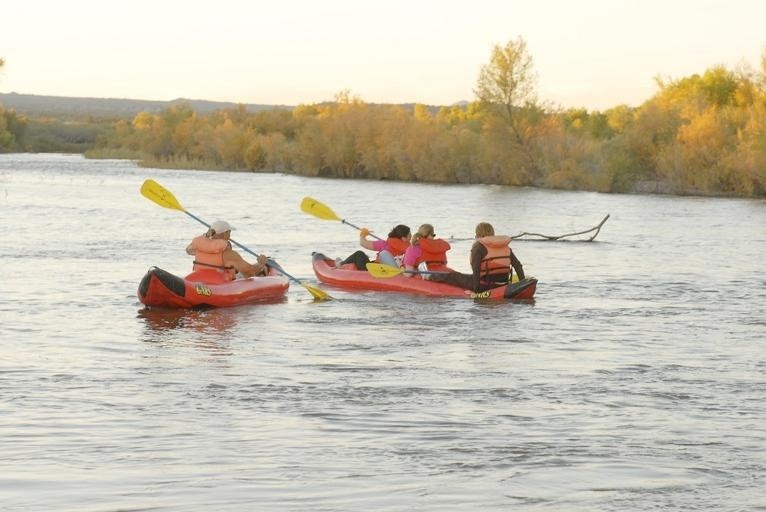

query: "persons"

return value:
[379, 226, 450, 275]
[186, 227, 266, 282]
[419, 224, 526, 294]
[334, 226, 412, 275]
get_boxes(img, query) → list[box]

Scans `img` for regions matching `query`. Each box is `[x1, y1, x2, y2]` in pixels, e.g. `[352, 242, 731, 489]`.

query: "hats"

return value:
[211, 220, 236, 233]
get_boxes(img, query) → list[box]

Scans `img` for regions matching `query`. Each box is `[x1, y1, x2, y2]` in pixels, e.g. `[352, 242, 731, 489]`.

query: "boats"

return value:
[312, 252, 538, 308]
[137, 255, 289, 307]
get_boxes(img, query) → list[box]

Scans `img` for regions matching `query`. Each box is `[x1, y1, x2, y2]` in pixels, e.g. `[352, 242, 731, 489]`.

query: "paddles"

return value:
[140, 178, 334, 301]
[367, 263, 451, 277]
[299, 197, 387, 242]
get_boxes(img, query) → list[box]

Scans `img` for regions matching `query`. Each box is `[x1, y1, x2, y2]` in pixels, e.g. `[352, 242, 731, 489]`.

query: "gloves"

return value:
[360, 228, 369, 237]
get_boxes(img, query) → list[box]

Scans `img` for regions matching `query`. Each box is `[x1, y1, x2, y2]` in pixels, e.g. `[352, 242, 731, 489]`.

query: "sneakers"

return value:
[334, 257, 344, 269]
[418, 262, 428, 279]
